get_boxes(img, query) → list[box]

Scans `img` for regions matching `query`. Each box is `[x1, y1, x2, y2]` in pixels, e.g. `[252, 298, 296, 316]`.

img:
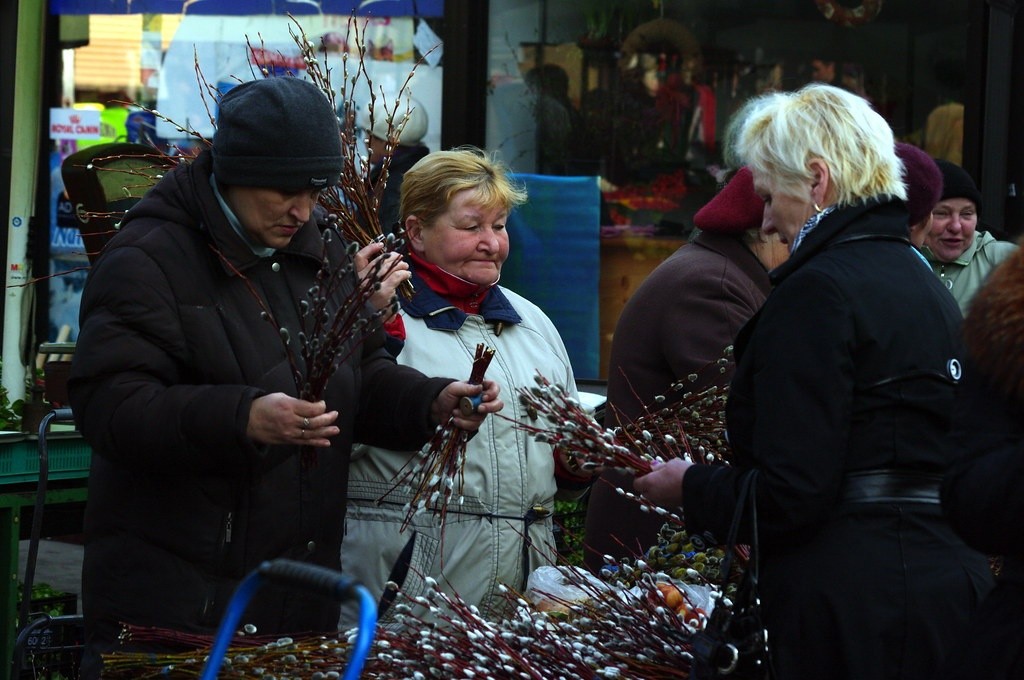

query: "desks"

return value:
[0, 487, 88, 680]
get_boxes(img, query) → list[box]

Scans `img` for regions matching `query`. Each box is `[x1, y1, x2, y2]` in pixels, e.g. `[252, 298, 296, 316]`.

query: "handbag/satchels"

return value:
[688, 467, 845, 680]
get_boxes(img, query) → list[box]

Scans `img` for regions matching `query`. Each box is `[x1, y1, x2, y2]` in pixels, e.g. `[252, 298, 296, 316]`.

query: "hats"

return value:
[932, 159, 982, 218]
[693, 165, 766, 235]
[892, 142, 944, 227]
[356, 94, 430, 149]
[210, 77, 345, 194]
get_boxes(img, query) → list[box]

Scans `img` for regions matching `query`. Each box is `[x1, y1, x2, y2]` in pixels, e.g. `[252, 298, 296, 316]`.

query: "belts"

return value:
[831, 465, 952, 507]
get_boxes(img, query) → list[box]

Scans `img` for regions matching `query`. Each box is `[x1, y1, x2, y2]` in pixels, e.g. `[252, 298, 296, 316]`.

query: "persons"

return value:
[356, 97, 430, 264]
[537, 66, 593, 179]
[339, 147, 606, 632]
[66, 77, 504, 680]
[634, 83, 995, 680]
[919, 159, 1018, 316]
[729, 52, 783, 116]
[813, 46, 864, 96]
[938, 241, 1024, 680]
[892, 143, 943, 251]
[582, 92, 792, 579]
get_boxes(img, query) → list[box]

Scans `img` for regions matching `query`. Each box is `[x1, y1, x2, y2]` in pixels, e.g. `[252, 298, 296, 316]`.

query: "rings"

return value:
[302, 430, 304, 438]
[304, 418, 309, 427]
